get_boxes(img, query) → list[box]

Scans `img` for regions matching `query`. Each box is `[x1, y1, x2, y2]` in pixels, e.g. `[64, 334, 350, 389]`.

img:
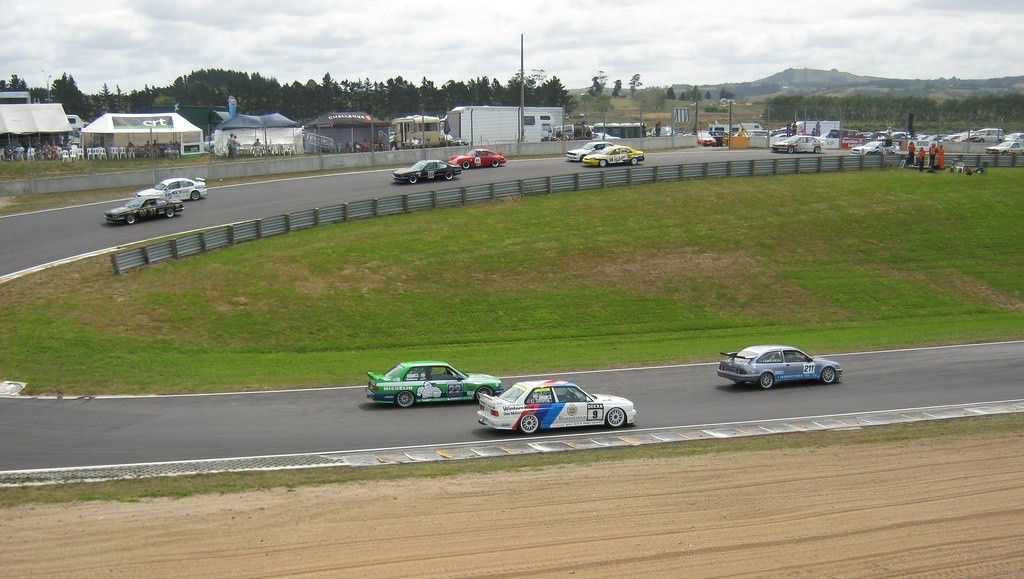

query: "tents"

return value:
[80, 112, 204, 160]
[304, 111, 390, 154]
[214, 113, 305, 156]
[0, 103, 72, 144]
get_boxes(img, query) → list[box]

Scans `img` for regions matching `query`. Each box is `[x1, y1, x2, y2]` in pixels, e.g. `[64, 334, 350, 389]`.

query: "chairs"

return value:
[0, 146, 180, 163]
[978, 162, 989, 171]
[323, 141, 421, 153]
[955, 162, 965, 173]
[222, 143, 297, 156]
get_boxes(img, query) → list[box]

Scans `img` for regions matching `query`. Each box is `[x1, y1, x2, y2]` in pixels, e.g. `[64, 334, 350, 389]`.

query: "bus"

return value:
[593, 123, 647, 137]
[393, 115, 445, 148]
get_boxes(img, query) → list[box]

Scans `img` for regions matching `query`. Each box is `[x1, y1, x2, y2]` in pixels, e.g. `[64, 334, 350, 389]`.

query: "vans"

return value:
[795, 121, 841, 136]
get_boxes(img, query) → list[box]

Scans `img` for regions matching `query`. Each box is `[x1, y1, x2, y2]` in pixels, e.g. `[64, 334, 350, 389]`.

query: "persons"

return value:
[231, 136, 238, 159]
[582, 118, 588, 124]
[597, 144, 602, 150]
[50, 142, 60, 159]
[363, 139, 369, 152]
[738, 129, 746, 136]
[144, 201, 151, 207]
[145, 140, 157, 151]
[254, 138, 262, 156]
[227, 134, 234, 157]
[787, 120, 821, 137]
[164, 140, 179, 158]
[35, 142, 46, 159]
[353, 141, 361, 153]
[5, 142, 14, 162]
[43, 142, 51, 159]
[14, 143, 24, 160]
[411, 138, 420, 149]
[885, 126, 944, 172]
[127, 141, 135, 147]
[642, 122, 647, 137]
[655, 121, 661, 136]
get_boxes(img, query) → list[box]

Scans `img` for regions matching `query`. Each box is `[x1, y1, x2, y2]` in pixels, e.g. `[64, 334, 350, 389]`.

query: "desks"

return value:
[401, 142, 414, 148]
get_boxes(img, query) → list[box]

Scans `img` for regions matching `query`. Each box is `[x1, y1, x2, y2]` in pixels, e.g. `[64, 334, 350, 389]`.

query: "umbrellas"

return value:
[444, 116, 451, 146]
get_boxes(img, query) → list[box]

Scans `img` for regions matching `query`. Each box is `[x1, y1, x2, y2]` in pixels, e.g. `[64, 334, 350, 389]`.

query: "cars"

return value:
[477, 380, 638, 436]
[365, 362, 503, 408]
[592, 133, 620, 139]
[742, 123, 764, 130]
[137, 177, 208, 201]
[652, 128, 729, 147]
[449, 148, 506, 170]
[771, 136, 826, 154]
[842, 127, 1024, 156]
[566, 142, 617, 163]
[392, 159, 463, 185]
[717, 344, 843, 389]
[105, 197, 185, 225]
[582, 146, 644, 168]
[746, 128, 792, 137]
